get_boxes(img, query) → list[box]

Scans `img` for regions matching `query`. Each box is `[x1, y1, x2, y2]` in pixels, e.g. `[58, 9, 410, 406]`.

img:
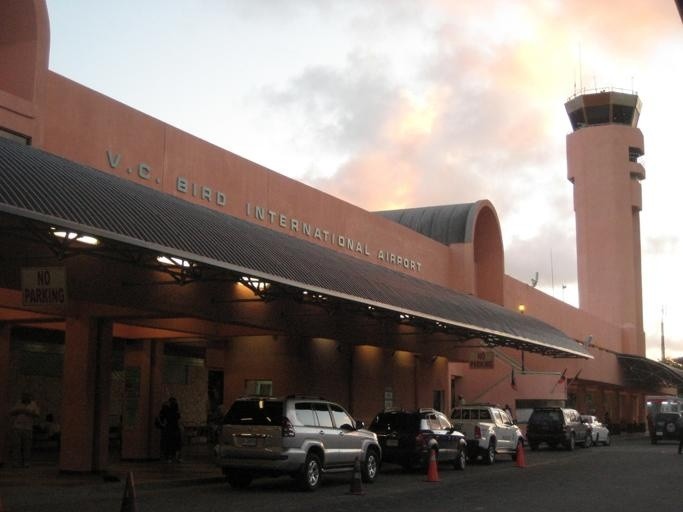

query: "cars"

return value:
[581, 414, 611, 446]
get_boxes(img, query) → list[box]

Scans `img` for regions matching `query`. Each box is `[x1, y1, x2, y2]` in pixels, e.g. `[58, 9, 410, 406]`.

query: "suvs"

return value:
[369, 408, 469, 472]
[650, 412, 682, 444]
[213, 392, 381, 493]
[525, 407, 592, 451]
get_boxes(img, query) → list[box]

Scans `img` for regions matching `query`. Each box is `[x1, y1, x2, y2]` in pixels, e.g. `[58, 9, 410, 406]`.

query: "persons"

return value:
[156, 397, 184, 460]
[8, 391, 40, 468]
[39, 412, 61, 441]
[458, 394, 466, 406]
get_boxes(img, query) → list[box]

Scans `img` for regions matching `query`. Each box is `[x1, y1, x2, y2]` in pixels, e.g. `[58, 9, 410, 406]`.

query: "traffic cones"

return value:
[422, 449, 443, 482]
[119, 470, 138, 512]
[344, 456, 366, 495]
[512, 440, 528, 468]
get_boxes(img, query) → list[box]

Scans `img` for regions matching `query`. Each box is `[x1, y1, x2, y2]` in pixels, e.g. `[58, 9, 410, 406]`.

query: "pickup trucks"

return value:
[447, 405, 525, 465]
